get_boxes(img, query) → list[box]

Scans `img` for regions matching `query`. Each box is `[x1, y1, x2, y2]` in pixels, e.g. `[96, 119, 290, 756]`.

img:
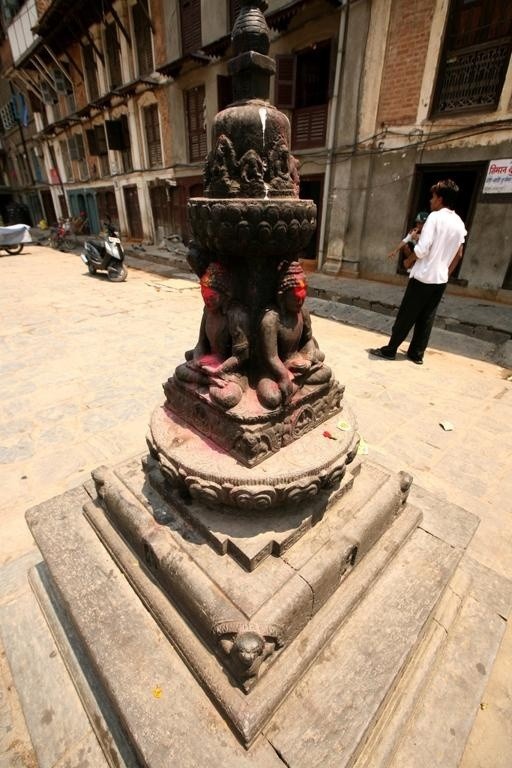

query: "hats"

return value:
[415, 212, 429, 222]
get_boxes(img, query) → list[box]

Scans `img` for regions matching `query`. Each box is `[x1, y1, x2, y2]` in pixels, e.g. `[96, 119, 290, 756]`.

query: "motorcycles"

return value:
[80, 212, 128, 282]
[0, 222, 33, 255]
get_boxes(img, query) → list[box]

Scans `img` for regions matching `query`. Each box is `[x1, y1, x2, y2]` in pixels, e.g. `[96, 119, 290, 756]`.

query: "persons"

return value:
[387, 212, 428, 276]
[370, 179, 467, 364]
[256, 262, 332, 409]
[39, 216, 48, 230]
[175, 262, 250, 409]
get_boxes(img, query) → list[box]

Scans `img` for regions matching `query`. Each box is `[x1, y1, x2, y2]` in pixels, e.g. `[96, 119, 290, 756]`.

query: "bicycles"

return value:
[48, 217, 79, 249]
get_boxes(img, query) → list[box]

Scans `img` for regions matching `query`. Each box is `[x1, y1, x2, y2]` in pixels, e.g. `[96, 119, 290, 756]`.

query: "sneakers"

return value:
[370, 349, 395, 360]
[396, 268, 410, 275]
[406, 351, 422, 364]
